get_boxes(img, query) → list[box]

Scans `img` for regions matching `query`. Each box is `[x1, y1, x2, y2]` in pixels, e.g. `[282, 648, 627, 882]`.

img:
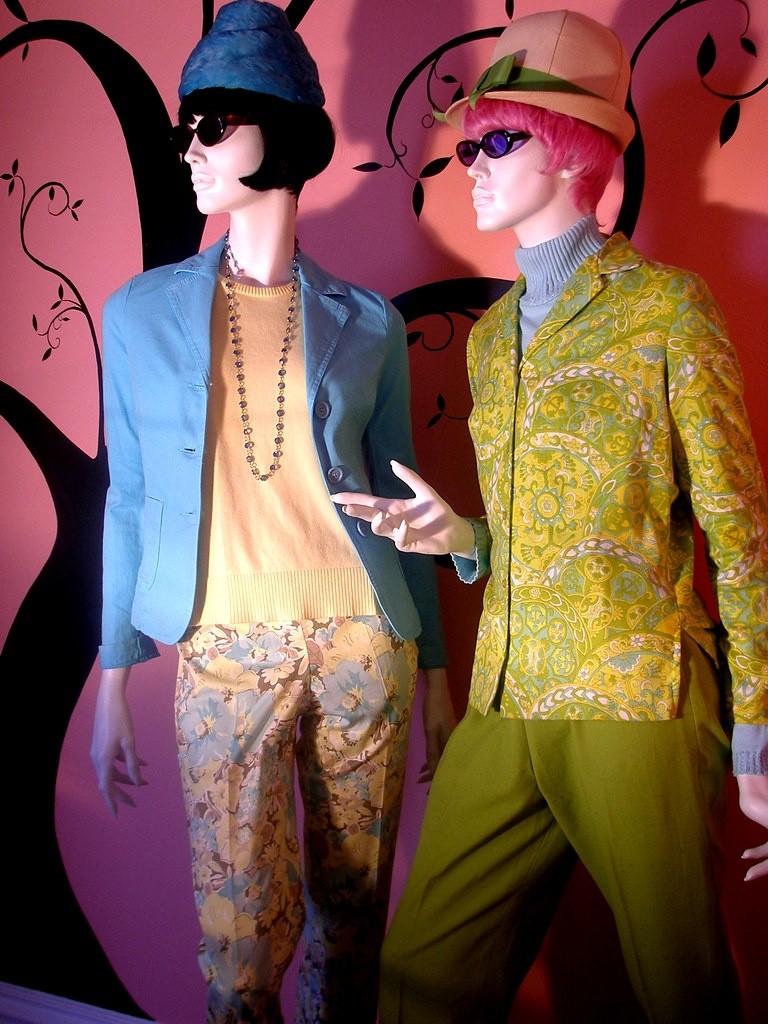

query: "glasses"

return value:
[456, 129, 530, 168]
[169, 116, 259, 153]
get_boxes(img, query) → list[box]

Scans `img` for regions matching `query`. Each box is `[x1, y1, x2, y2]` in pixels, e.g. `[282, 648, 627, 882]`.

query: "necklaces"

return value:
[222, 228, 299, 484]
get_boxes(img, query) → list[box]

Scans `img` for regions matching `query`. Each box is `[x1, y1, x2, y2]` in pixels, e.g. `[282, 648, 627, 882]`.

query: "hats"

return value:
[442, 9, 636, 157]
[179, 0, 325, 108]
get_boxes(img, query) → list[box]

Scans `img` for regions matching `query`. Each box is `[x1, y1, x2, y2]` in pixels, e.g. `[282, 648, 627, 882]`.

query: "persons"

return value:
[323, 9, 767, 1024]
[87, 2, 460, 1024]
